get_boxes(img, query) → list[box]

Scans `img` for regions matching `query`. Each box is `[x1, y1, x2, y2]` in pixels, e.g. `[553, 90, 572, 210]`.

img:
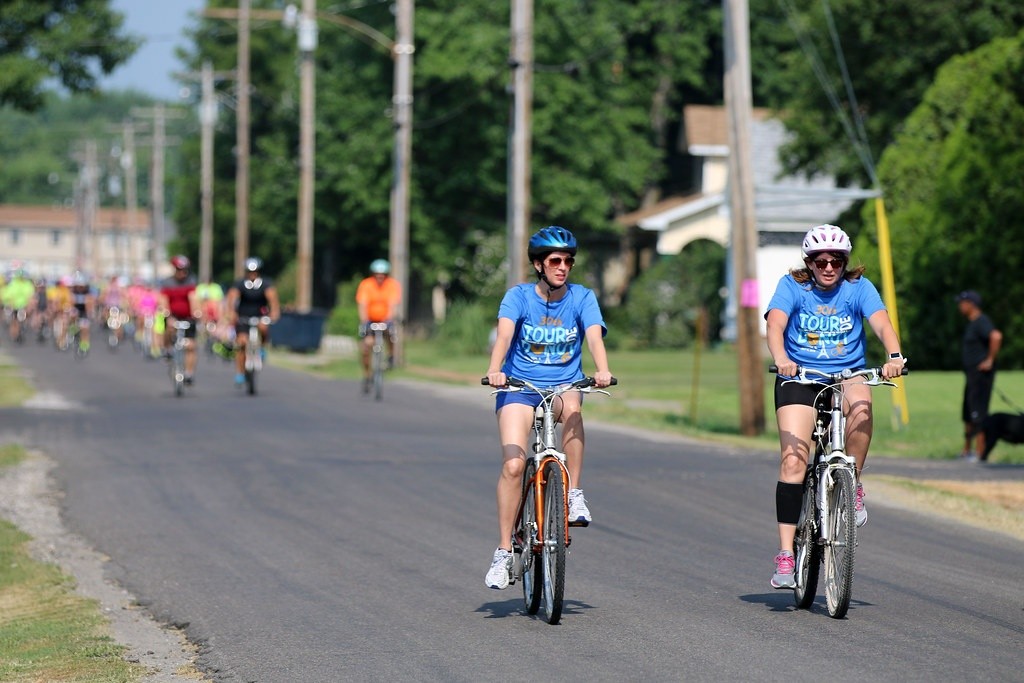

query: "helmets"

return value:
[369, 259, 390, 275]
[172, 255, 190, 270]
[801, 225, 851, 261]
[244, 256, 262, 272]
[529, 227, 578, 262]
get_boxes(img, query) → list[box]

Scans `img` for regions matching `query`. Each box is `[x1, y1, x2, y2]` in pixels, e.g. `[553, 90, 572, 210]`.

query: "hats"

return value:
[954, 291, 982, 305]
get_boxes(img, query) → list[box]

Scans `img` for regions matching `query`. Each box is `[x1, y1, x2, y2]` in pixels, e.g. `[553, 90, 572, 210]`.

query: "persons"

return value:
[0, 267, 169, 359]
[194, 271, 223, 357]
[355, 259, 402, 395]
[224, 257, 279, 384]
[957, 289, 1003, 466]
[485, 227, 612, 590]
[763, 223, 903, 590]
[160, 255, 201, 387]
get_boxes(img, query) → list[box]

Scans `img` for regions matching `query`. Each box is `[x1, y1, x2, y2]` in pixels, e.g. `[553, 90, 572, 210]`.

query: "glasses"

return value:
[810, 258, 845, 270]
[542, 257, 577, 268]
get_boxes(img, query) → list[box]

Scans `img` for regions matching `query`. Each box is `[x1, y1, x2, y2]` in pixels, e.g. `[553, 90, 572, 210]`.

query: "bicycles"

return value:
[361, 320, 395, 402]
[234, 315, 279, 394]
[161, 319, 203, 398]
[768, 362, 910, 620]
[481, 374, 618, 626]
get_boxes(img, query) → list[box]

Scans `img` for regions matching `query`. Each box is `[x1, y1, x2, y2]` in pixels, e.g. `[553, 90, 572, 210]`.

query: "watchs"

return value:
[888, 352, 907, 368]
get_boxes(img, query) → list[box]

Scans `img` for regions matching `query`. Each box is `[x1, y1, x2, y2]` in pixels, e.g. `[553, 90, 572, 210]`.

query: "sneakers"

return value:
[842, 482, 867, 528]
[567, 488, 591, 524]
[485, 548, 515, 590]
[771, 549, 797, 589]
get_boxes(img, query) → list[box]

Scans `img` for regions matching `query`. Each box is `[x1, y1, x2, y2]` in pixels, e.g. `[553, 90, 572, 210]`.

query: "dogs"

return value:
[964, 412, 1024, 460]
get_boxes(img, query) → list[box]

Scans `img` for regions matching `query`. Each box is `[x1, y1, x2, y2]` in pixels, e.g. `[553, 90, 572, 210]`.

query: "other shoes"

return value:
[258, 346, 267, 360]
[234, 374, 244, 384]
